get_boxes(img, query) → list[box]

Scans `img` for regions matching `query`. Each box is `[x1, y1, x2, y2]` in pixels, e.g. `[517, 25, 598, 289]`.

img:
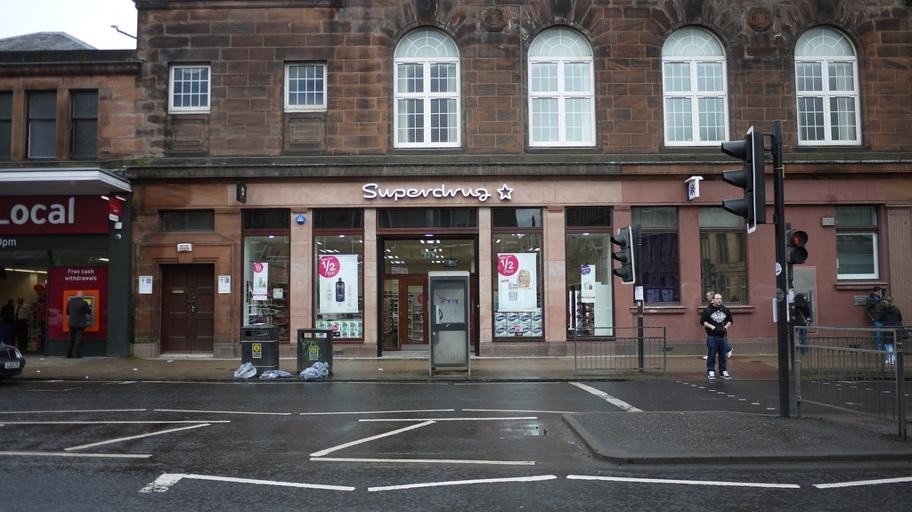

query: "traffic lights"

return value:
[784, 228, 809, 267]
[608, 225, 638, 286]
[717, 124, 767, 235]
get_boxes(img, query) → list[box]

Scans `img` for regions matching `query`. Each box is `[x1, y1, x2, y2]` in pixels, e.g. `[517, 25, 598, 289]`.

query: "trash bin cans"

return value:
[240, 323, 279, 377]
[297, 328, 333, 377]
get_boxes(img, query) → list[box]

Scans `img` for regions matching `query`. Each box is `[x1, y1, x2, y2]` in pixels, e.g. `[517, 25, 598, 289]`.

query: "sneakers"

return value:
[720, 370, 731, 380]
[727, 347, 733, 358]
[67, 354, 83, 358]
[703, 355, 708, 360]
[707, 371, 716, 380]
[885, 359, 895, 364]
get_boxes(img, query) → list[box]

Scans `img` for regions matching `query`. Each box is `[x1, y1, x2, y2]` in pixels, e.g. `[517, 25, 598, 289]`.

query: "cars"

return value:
[0, 342, 26, 381]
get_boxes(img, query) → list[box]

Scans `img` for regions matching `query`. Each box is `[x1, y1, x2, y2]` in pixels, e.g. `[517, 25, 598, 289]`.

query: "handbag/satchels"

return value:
[806, 321, 817, 332]
[901, 328, 908, 339]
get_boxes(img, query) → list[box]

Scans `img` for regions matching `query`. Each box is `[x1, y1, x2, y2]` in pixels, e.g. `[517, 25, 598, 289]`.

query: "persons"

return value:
[876, 295, 902, 366]
[0, 298, 16, 350]
[789, 294, 812, 354]
[699, 291, 734, 360]
[864, 286, 886, 350]
[64, 289, 92, 359]
[12, 295, 33, 352]
[517, 269, 531, 289]
[699, 293, 733, 381]
